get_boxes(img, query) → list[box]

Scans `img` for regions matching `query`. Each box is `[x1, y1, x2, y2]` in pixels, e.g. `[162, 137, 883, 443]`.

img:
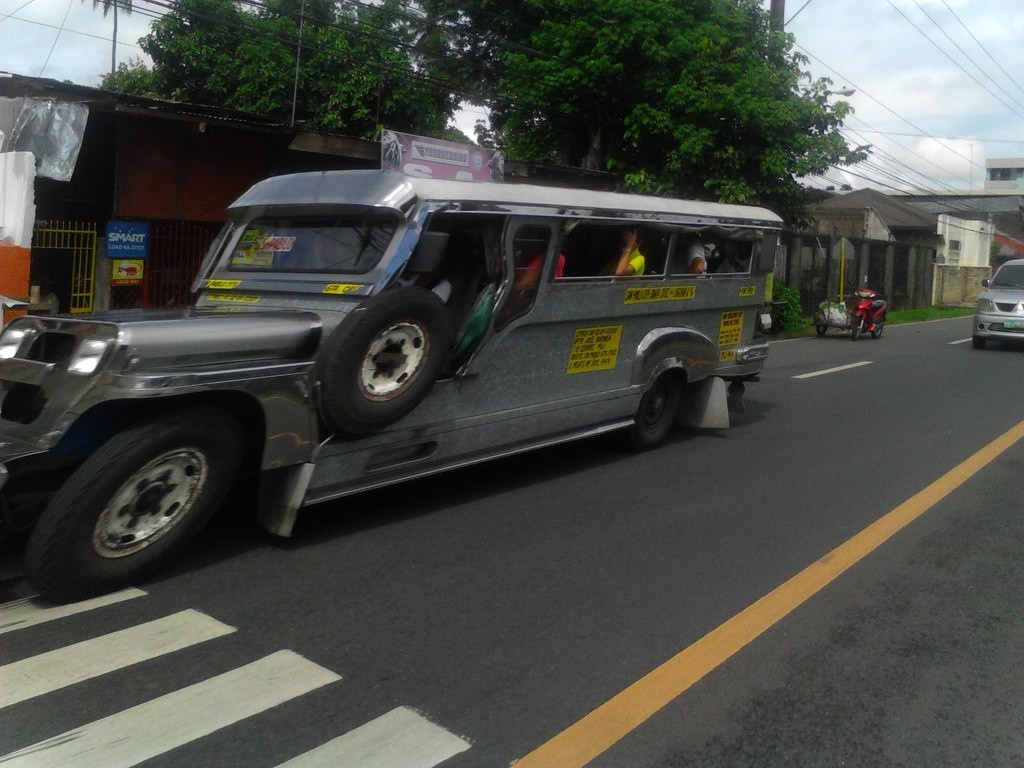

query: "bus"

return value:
[0, 170, 784, 603]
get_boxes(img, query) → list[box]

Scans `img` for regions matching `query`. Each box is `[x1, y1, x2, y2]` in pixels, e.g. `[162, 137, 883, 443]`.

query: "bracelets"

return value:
[622, 248, 632, 254]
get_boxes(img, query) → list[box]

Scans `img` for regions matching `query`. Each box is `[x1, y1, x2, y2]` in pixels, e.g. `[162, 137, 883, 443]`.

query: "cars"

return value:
[973, 259, 1024, 347]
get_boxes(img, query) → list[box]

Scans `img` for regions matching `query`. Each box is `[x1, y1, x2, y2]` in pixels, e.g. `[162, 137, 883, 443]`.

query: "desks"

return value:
[0, 298, 55, 326]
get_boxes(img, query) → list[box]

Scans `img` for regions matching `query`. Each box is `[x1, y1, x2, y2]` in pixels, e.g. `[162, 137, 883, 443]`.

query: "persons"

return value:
[513, 229, 565, 291]
[604, 227, 646, 280]
[716, 243, 751, 273]
[686, 243, 706, 277]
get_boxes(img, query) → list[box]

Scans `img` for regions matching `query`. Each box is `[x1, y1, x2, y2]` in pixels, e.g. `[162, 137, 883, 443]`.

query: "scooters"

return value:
[849, 288, 887, 341]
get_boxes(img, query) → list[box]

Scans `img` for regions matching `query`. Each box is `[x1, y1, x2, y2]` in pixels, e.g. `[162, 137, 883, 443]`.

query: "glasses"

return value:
[635, 236, 642, 241]
